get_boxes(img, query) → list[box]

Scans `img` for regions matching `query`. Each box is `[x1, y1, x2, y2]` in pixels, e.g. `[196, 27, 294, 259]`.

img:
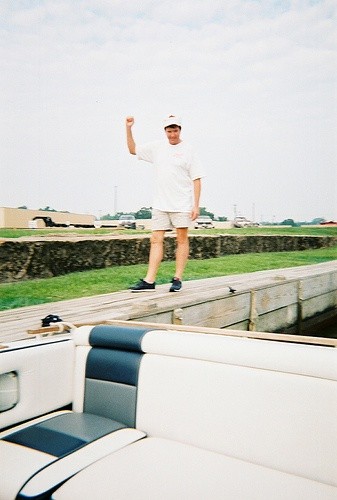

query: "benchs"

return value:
[0, 325, 336, 500]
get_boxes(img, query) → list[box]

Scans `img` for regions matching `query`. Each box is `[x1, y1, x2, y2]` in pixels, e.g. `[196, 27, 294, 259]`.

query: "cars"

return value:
[195, 215, 213, 229]
[234, 215, 259, 228]
[119, 214, 136, 228]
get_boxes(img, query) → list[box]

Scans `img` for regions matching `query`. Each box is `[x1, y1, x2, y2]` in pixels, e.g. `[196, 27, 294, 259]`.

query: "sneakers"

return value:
[169, 277, 183, 292]
[130, 278, 155, 291]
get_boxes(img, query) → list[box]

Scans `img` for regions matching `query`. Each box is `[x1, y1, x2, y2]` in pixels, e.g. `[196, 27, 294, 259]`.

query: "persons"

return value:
[126, 115, 205, 292]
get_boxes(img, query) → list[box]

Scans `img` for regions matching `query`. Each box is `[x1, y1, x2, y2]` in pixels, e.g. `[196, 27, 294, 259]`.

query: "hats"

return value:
[164, 115, 181, 129]
